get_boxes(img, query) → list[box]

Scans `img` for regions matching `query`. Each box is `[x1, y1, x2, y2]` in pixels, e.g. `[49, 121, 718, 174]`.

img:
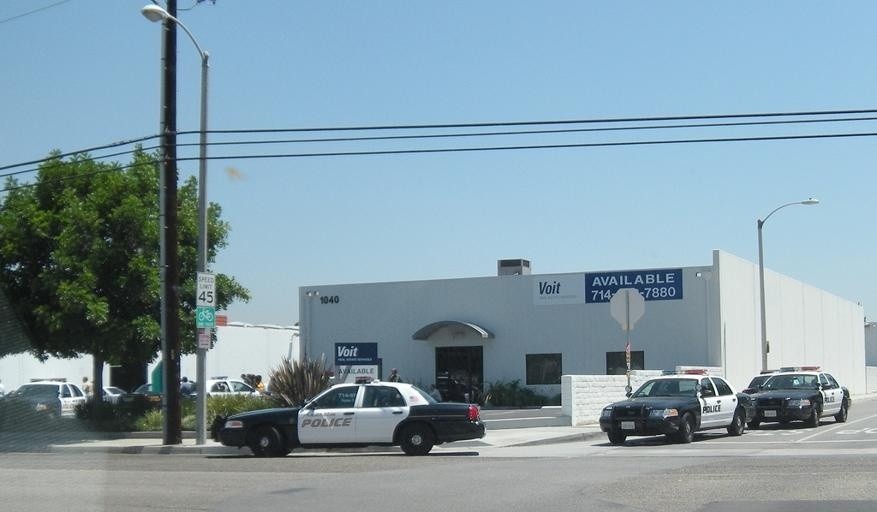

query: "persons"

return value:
[82, 376, 93, 393]
[256, 375, 264, 391]
[429, 383, 442, 402]
[180, 376, 190, 389]
[0, 379, 5, 398]
[386, 368, 403, 382]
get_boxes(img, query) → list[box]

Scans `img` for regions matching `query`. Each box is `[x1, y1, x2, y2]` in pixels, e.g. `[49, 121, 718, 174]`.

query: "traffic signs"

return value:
[196, 272, 215, 306]
[196, 306, 215, 329]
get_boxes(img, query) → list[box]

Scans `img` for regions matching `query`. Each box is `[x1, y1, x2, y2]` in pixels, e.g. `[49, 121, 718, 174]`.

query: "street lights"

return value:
[141, 5, 209, 447]
[758, 198, 818, 371]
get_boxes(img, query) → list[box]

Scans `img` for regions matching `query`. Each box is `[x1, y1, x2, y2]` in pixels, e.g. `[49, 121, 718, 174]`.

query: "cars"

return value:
[0, 379, 87, 421]
[102, 376, 263, 416]
[220, 380, 484, 455]
[599, 370, 754, 442]
[742, 367, 851, 429]
